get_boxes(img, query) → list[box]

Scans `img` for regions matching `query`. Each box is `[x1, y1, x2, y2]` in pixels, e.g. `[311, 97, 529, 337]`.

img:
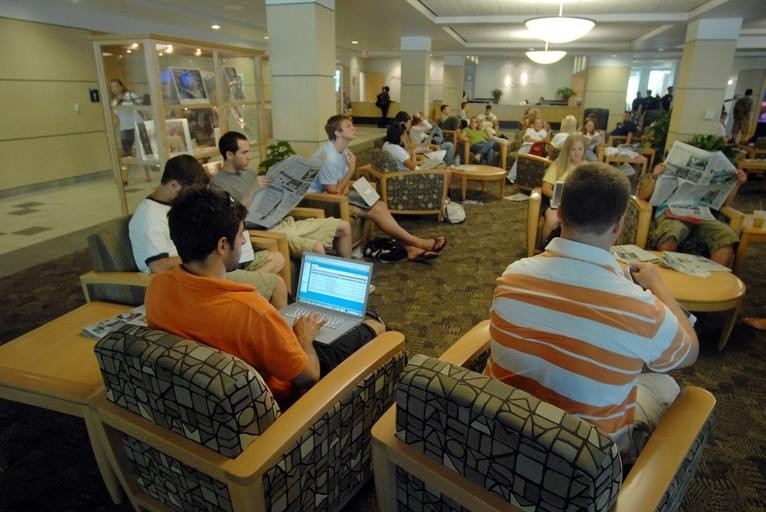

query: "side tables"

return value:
[0, 300, 137, 505]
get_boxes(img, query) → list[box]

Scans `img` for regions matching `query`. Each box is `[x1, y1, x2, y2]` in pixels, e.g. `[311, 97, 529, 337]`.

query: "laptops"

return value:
[549, 181, 564, 209]
[346, 176, 382, 211]
[272, 249, 374, 346]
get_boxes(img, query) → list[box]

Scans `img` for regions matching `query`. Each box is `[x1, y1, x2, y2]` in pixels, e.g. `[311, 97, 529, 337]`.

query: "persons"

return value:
[715, 88, 764, 158]
[109, 78, 151, 187]
[127, 154, 288, 312]
[343, 91, 353, 108]
[306, 114, 448, 263]
[636, 161, 747, 330]
[373, 85, 674, 202]
[144, 183, 388, 419]
[209, 131, 377, 296]
[486, 161, 701, 481]
[541, 134, 594, 251]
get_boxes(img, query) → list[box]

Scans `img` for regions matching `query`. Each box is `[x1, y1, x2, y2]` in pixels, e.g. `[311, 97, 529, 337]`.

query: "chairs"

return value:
[298, 177, 377, 254]
[80, 212, 289, 307]
[370, 320, 717, 512]
[203, 161, 324, 298]
[87, 319, 407, 512]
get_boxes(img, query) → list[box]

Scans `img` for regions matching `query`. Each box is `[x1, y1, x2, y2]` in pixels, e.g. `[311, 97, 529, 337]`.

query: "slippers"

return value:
[408, 237, 446, 261]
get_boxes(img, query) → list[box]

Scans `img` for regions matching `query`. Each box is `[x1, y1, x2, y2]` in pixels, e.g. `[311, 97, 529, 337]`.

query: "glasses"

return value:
[221, 190, 235, 234]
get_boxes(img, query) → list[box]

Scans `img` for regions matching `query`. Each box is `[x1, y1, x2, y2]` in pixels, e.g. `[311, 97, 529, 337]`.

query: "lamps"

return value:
[522, 3, 596, 44]
[524, 41, 568, 65]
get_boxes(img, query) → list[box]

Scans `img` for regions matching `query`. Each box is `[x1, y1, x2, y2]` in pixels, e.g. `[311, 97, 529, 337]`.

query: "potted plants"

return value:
[555, 86, 577, 103]
[490, 88, 503, 103]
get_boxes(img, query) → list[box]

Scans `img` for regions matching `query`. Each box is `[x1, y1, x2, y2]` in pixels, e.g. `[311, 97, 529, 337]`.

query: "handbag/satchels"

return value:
[363, 238, 407, 261]
[443, 199, 466, 223]
[530, 142, 544, 156]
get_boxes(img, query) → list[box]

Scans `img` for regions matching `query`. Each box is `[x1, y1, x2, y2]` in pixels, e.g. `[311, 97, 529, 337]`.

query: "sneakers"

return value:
[506, 176, 514, 184]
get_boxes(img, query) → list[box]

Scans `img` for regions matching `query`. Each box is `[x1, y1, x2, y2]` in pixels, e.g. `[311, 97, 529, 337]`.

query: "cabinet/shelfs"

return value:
[88, 33, 265, 217]
